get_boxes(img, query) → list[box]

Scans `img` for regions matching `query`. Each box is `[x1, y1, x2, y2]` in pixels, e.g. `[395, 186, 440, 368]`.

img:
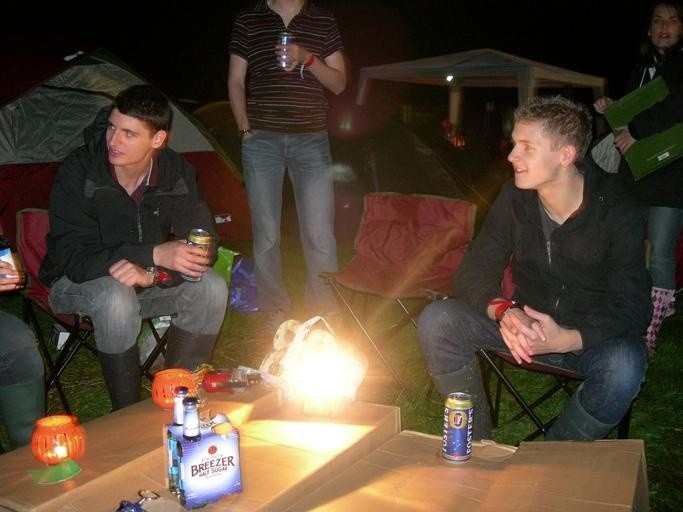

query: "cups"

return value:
[201, 369, 267, 392]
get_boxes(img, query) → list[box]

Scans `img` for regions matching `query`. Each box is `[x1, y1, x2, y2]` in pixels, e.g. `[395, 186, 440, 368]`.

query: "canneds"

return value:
[179, 228, 211, 282]
[442, 392, 475, 461]
[275, 32, 296, 68]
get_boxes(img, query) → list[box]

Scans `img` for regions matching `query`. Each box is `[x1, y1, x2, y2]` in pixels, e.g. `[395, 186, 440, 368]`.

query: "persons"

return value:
[591, 0, 683, 355]
[1, 228, 48, 452]
[416, 92, 655, 441]
[227, 0, 348, 331]
[37, 82, 229, 414]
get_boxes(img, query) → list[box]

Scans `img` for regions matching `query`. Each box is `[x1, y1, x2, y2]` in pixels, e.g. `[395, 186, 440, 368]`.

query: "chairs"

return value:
[9, 201, 178, 425]
[472, 200, 680, 443]
[314, 187, 482, 394]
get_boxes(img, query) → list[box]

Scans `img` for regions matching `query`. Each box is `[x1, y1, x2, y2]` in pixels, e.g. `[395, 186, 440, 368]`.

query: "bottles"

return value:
[167, 385, 188, 496]
[0, 226, 26, 287]
[177, 397, 201, 508]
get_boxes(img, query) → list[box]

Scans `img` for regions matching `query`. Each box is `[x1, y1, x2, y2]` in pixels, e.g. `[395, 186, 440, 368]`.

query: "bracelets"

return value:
[488, 298, 520, 328]
[239, 129, 251, 137]
[303, 54, 314, 68]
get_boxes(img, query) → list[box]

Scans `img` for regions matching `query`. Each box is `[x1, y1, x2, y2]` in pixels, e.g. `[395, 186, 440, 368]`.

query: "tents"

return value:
[1, 46, 297, 244]
[195, 100, 492, 301]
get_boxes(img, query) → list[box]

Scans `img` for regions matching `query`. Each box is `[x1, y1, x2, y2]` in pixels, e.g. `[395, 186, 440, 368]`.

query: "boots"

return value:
[545, 380, 622, 443]
[645, 285, 676, 357]
[162, 320, 219, 373]
[0, 373, 47, 448]
[94, 341, 143, 413]
[427, 355, 494, 441]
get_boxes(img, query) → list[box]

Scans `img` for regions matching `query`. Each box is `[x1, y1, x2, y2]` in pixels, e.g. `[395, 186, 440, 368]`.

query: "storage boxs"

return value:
[65, 394, 404, 510]
[289, 420, 518, 512]
[1, 362, 286, 512]
[475, 437, 652, 512]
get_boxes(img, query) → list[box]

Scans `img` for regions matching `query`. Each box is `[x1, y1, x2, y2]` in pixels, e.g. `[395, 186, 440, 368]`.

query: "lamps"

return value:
[150, 366, 201, 414]
[27, 411, 87, 466]
